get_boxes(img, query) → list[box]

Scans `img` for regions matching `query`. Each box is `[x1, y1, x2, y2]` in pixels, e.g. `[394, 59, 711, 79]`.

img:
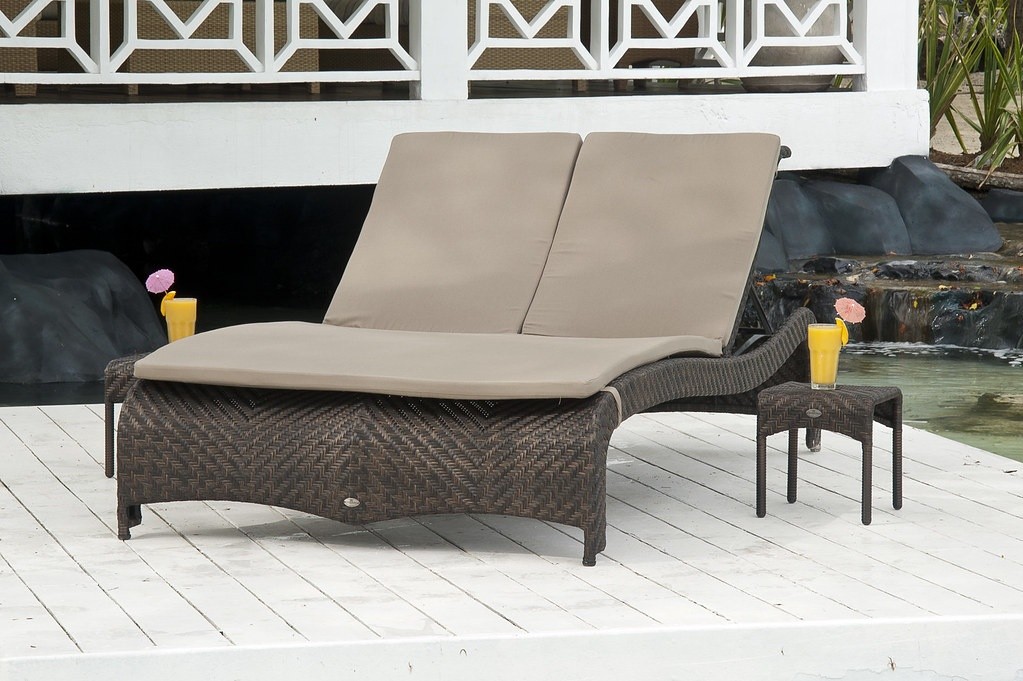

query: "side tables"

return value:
[103, 352, 153, 479]
[755, 380, 905, 527]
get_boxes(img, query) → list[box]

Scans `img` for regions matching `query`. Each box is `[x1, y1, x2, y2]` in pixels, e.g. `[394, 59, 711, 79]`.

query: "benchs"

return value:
[115, 131, 822, 570]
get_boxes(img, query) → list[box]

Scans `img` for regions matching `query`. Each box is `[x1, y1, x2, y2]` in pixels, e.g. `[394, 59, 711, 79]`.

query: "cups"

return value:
[807, 324, 841, 390]
[165, 298, 197, 343]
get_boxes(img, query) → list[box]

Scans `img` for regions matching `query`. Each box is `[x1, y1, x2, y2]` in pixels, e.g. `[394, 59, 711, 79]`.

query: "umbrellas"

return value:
[833, 296, 866, 324]
[145, 269, 175, 295]
